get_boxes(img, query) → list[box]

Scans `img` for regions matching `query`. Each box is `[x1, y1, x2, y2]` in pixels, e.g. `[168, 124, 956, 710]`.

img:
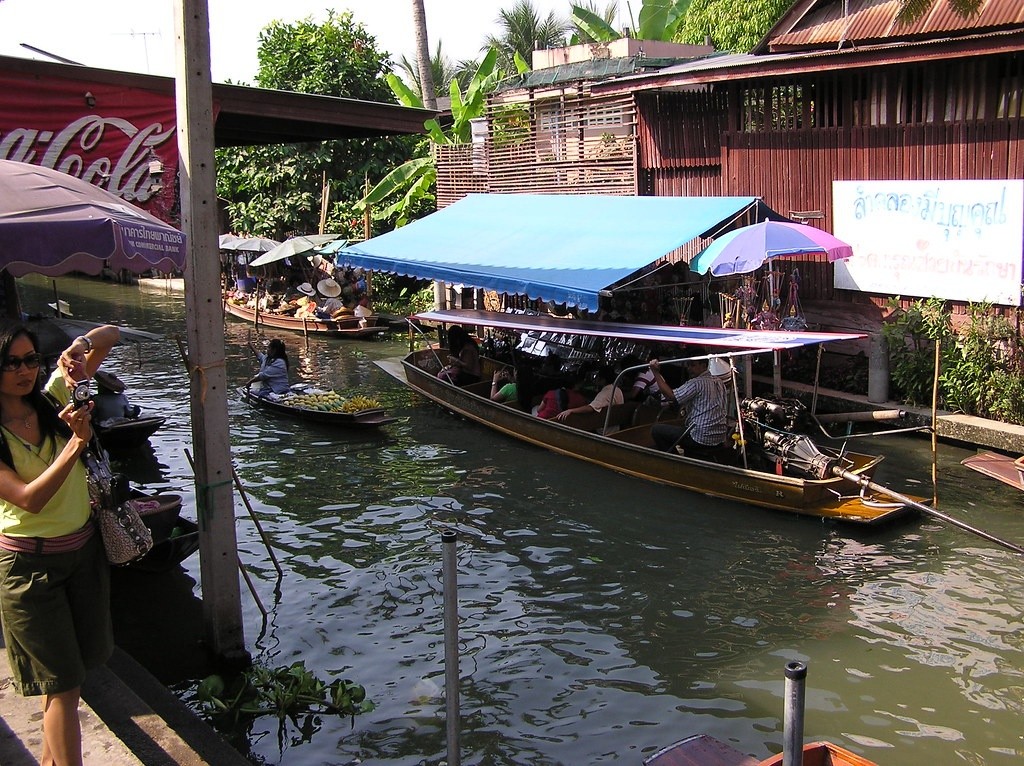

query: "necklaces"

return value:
[24, 417, 31, 429]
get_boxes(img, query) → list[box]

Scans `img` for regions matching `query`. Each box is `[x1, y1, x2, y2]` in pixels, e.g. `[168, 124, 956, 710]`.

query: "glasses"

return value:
[0, 353, 42, 372]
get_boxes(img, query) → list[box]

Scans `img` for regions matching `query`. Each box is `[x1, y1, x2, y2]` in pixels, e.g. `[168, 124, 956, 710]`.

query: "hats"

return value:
[353, 305, 373, 318]
[708, 358, 731, 383]
[313, 254, 323, 268]
[95, 369, 125, 392]
[333, 306, 354, 320]
[297, 282, 316, 296]
[317, 279, 341, 297]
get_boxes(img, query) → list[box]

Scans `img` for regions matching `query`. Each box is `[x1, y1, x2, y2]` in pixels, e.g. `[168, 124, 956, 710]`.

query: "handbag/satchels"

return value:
[40, 388, 154, 567]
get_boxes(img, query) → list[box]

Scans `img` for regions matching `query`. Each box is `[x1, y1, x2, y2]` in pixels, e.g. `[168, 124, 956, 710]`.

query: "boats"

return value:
[750, 739, 880, 766]
[137, 276, 185, 301]
[372, 307, 937, 529]
[221, 277, 388, 335]
[237, 380, 400, 427]
[47, 367, 201, 575]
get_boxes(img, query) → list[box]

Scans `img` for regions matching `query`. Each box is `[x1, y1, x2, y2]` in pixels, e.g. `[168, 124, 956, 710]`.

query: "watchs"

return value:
[492, 382, 497, 385]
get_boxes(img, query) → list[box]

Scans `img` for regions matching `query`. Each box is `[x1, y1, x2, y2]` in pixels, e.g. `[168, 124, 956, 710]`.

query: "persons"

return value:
[0, 318, 119, 766]
[437, 325, 480, 385]
[93, 371, 140, 419]
[556, 366, 624, 435]
[620, 355, 667, 402]
[490, 353, 586, 420]
[246, 339, 291, 394]
[648, 350, 727, 460]
[46, 300, 73, 318]
[297, 256, 367, 307]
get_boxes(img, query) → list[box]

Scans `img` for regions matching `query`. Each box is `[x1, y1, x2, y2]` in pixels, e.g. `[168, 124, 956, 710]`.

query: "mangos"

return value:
[284, 390, 346, 411]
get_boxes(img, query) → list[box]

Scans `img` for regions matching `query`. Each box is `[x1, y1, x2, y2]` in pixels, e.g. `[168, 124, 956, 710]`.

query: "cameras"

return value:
[72, 379, 89, 411]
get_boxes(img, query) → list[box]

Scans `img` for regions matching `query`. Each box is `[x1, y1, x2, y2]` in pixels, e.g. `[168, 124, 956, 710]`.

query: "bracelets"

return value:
[81, 336, 91, 354]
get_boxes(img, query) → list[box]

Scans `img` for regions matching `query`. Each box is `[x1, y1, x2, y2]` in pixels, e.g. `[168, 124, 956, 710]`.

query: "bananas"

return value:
[332, 394, 381, 413]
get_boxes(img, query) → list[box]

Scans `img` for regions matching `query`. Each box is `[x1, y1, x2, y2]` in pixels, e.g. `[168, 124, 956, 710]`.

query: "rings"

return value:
[78, 418, 83, 421]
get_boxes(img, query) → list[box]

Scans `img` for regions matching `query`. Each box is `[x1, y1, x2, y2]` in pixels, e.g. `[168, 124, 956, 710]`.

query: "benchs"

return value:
[457, 352, 686, 446]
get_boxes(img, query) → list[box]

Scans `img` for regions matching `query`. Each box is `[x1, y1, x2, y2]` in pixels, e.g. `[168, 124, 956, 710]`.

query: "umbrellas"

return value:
[219, 234, 237, 245]
[220, 236, 283, 252]
[0, 155, 186, 317]
[691, 217, 852, 311]
[249, 233, 341, 282]
